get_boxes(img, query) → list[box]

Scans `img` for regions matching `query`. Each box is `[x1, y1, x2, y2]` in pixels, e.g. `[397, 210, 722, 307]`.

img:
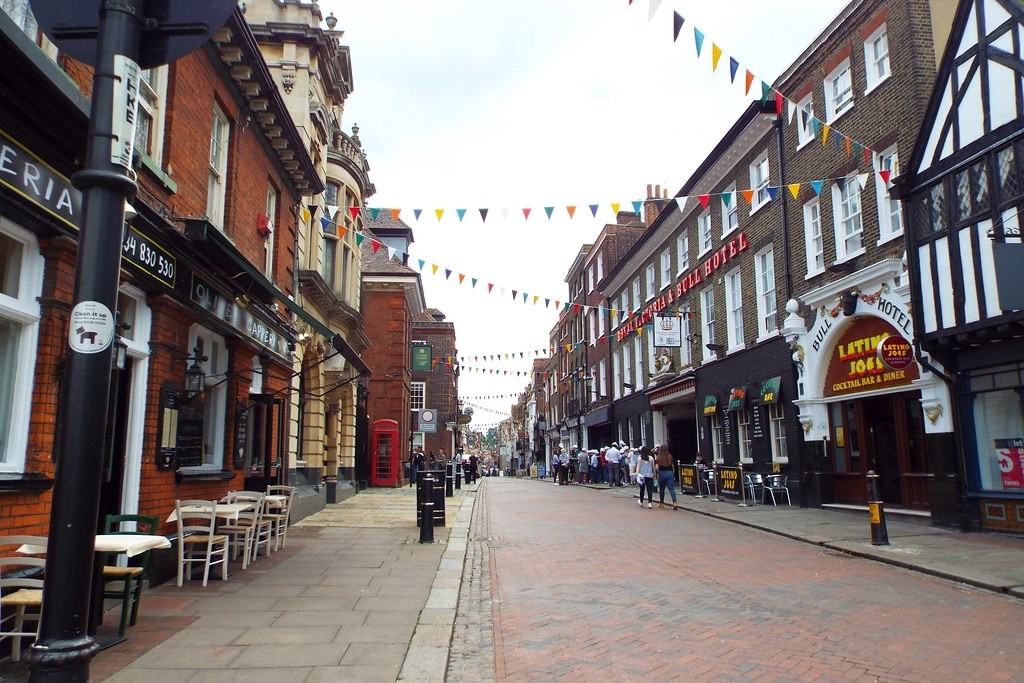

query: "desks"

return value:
[15, 535, 171, 653]
[223, 495, 286, 506]
[166, 505, 252, 581]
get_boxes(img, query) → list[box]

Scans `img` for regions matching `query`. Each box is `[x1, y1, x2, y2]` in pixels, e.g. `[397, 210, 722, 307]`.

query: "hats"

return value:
[572, 445, 578, 449]
[418, 444, 422, 448]
[582, 440, 660, 454]
[559, 443, 564, 448]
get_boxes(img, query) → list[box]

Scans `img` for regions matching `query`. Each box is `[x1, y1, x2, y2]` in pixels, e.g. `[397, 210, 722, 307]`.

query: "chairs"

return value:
[743, 472, 791, 506]
[217, 490, 274, 570]
[702, 469, 717, 494]
[0, 535, 50, 662]
[175, 498, 230, 586]
[260, 484, 295, 552]
[98, 515, 161, 635]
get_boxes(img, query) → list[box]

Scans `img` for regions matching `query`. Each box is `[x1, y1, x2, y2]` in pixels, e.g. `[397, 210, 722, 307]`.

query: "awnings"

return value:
[169, 214, 374, 397]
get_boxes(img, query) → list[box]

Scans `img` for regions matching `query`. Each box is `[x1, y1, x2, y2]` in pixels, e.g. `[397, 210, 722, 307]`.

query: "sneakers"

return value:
[647, 503, 652, 509]
[638, 500, 643, 506]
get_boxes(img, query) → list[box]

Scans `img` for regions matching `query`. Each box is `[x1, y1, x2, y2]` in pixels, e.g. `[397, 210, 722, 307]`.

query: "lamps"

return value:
[170, 347, 210, 400]
[228, 271, 254, 308]
[622, 383, 635, 389]
[707, 344, 723, 351]
[827, 264, 851, 273]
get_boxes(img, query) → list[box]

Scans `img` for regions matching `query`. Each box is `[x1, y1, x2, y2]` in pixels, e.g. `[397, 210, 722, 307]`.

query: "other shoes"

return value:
[672, 502, 678, 510]
[553, 480, 641, 487]
[657, 503, 665, 508]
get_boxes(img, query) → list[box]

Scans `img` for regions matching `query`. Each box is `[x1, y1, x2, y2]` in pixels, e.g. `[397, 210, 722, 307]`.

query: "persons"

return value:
[553, 441, 677, 510]
[691, 452, 709, 492]
[505, 466, 509, 476]
[520, 460, 536, 476]
[409, 445, 478, 484]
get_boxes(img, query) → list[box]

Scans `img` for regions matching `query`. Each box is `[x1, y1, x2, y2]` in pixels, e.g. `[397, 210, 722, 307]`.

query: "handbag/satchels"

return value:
[652, 479, 658, 488]
[476, 470, 481, 478]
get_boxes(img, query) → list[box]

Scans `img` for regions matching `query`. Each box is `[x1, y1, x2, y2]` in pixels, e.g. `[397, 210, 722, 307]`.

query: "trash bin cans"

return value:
[416, 470, 446, 527]
